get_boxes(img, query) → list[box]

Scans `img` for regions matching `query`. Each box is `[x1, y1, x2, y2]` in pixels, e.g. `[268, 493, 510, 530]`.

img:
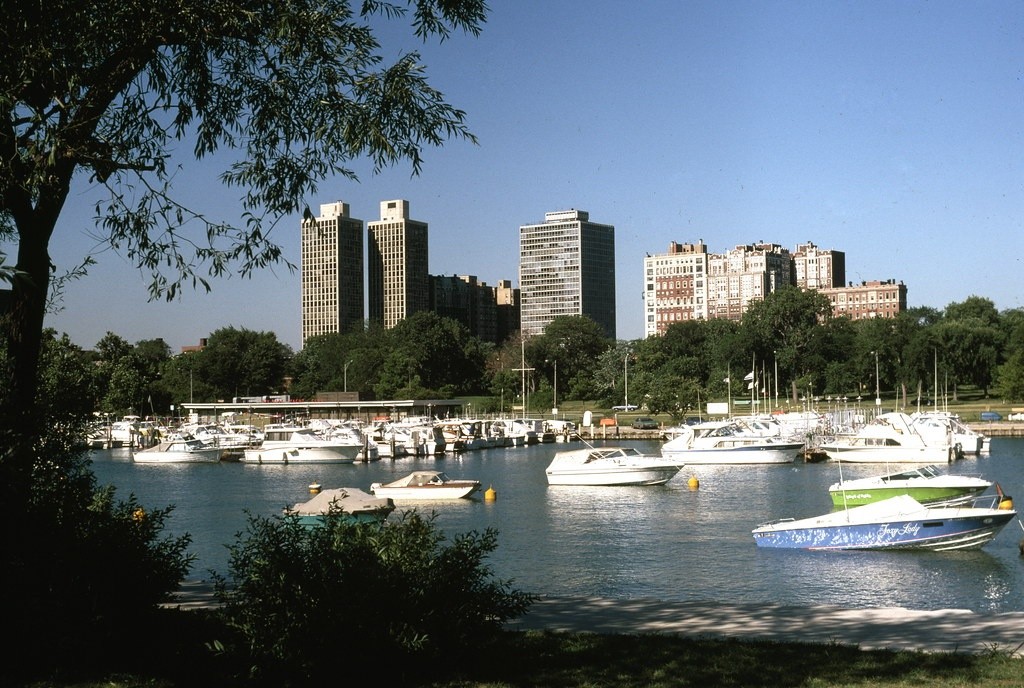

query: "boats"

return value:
[828, 465, 994, 507]
[659, 406, 991, 464]
[546, 447, 686, 490]
[750, 495, 1017, 552]
[87, 417, 578, 464]
[281, 488, 394, 535]
[375, 470, 478, 501]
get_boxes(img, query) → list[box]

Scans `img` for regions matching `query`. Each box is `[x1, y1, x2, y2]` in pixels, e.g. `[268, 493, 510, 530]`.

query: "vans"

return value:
[979, 411, 1003, 421]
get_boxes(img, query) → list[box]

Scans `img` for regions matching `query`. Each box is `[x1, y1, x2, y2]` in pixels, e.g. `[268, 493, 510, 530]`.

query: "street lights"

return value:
[344, 359, 353, 393]
[545, 355, 557, 409]
[870, 350, 879, 405]
[772, 351, 779, 406]
[624, 349, 635, 411]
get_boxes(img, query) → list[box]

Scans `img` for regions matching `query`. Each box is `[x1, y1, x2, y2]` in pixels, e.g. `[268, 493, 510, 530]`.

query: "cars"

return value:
[684, 416, 704, 425]
[611, 403, 638, 412]
[911, 397, 935, 407]
[629, 418, 659, 430]
